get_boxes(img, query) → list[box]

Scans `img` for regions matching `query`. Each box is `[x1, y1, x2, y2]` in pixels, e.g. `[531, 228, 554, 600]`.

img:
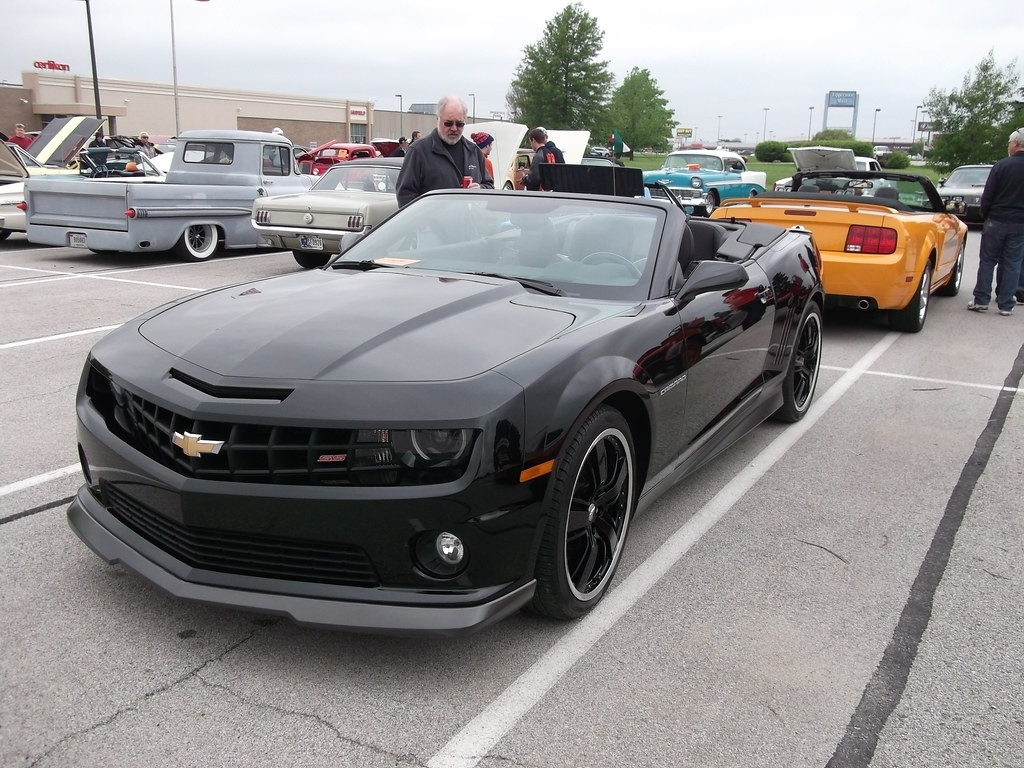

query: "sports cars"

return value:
[0, 147, 163, 240]
[67, 164, 824, 632]
[709, 169, 969, 333]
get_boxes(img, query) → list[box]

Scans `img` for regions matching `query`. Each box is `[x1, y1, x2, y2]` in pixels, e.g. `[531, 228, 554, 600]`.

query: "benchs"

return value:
[548, 213, 728, 277]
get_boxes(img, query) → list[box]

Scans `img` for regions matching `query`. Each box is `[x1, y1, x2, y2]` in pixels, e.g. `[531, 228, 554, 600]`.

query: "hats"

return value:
[471, 132, 494, 149]
[399, 137, 407, 144]
[93, 132, 104, 136]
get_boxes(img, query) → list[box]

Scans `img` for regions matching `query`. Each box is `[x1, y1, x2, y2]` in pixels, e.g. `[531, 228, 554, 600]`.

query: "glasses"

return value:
[1014, 127, 1022, 135]
[142, 136, 149, 138]
[440, 120, 465, 127]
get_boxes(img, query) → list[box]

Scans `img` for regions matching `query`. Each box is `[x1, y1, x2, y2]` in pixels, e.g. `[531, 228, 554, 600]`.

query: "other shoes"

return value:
[1013, 291, 1024, 305]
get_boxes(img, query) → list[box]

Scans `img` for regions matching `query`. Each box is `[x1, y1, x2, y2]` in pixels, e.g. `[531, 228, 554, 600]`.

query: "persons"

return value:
[409, 130, 422, 145]
[516, 126, 565, 192]
[134, 132, 157, 160]
[88, 131, 107, 147]
[471, 132, 495, 180]
[395, 95, 495, 209]
[8, 123, 33, 150]
[389, 137, 409, 157]
[967, 128, 1024, 316]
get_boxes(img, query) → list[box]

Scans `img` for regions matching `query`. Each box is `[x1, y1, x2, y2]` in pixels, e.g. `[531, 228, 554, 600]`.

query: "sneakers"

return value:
[998, 296, 1017, 315]
[967, 300, 989, 312]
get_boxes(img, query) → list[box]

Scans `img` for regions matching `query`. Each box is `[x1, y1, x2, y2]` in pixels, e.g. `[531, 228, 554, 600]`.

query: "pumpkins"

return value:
[126, 162, 138, 171]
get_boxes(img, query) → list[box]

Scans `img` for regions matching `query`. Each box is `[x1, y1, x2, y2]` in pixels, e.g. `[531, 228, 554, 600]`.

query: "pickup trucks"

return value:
[25, 128, 322, 262]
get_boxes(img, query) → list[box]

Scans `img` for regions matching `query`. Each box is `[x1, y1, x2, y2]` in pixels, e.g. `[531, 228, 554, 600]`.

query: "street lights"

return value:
[764, 108, 770, 141]
[913, 105, 927, 144]
[395, 95, 402, 137]
[169, 0, 209, 136]
[468, 94, 475, 123]
[872, 109, 881, 142]
[808, 107, 814, 140]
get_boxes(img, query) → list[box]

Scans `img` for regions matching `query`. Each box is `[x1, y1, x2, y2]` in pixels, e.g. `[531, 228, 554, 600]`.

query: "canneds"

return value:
[463, 176, 473, 189]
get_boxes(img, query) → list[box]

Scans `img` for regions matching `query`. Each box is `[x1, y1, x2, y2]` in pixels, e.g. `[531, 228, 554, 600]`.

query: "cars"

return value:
[591, 146, 612, 157]
[774, 146, 890, 198]
[250, 121, 529, 269]
[545, 129, 621, 168]
[299, 143, 377, 176]
[6, 116, 107, 171]
[937, 164, 996, 224]
[873, 146, 893, 160]
[643, 150, 767, 218]
